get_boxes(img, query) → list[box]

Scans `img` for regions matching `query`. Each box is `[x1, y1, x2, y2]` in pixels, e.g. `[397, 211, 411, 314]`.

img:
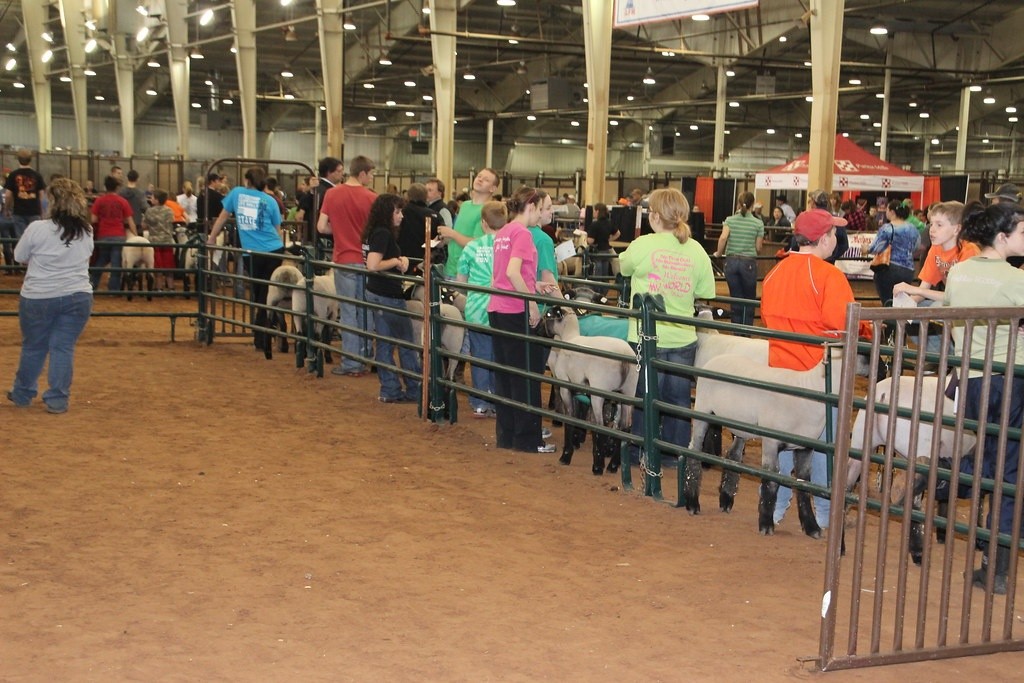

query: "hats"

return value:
[794, 211, 848, 243]
[208, 174, 223, 183]
[985, 183, 1021, 203]
[775, 195, 788, 202]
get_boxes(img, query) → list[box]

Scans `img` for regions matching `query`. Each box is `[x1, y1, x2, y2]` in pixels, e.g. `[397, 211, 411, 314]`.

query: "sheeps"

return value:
[690, 304, 770, 454]
[174, 229, 198, 300]
[544, 287, 638, 475]
[848, 375, 976, 502]
[682, 347, 887, 540]
[122, 227, 154, 301]
[264, 250, 466, 383]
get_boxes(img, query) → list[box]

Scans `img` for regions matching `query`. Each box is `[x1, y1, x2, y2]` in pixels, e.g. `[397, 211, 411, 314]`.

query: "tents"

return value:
[750, 131, 930, 214]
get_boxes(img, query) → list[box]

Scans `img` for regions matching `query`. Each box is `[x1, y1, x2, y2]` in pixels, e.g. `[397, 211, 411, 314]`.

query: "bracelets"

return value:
[210, 237, 216, 240]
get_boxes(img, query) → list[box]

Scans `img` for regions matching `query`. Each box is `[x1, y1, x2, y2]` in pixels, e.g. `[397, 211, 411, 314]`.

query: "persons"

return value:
[206, 168, 289, 351]
[264, 175, 311, 242]
[309, 158, 346, 341]
[317, 156, 382, 376]
[7, 177, 96, 413]
[47, 166, 245, 302]
[435, 167, 575, 453]
[364, 178, 453, 403]
[0, 149, 47, 276]
[712, 191, 765, 339]
[359, 176, 937, 307]
[606, 187, 718, 471]
[890, 176, 1024, 596]
[892, 200, 983, 376]
[760, 207, 887, 529]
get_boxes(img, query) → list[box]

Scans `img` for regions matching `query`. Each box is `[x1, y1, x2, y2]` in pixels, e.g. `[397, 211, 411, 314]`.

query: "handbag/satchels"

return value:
[868, 226, 895, 272]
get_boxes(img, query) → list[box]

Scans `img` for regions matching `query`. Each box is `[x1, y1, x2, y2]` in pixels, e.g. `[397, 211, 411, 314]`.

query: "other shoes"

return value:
[537, 442, 556, 453]
[474, 407, 497, 418]
[332, 366, 365, 376]
[6, 391, 15, 403]
[963, 568, 1008, 595]
[541, 427, 551, 438]
[890, 454, 930, 506]
[379, 392, 418, 404]
